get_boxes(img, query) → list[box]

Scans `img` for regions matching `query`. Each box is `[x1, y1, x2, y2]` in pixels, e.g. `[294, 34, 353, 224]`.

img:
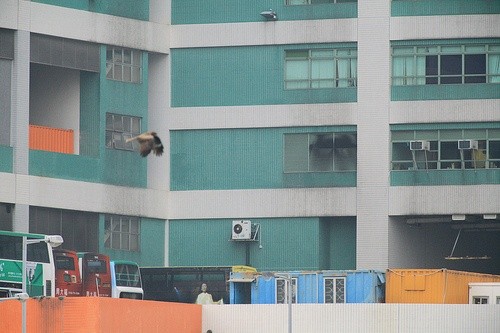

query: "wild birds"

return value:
[122, 130, 165, 158]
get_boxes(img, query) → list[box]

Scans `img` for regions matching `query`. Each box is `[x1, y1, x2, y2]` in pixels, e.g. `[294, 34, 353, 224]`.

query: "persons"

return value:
[196, 283, 212, 303]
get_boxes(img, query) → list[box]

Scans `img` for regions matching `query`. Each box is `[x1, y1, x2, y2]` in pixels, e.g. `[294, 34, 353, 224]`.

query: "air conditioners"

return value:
[231, 219, 251, 239]
[410, 139, 429, 151]
[457, 138, 475, 150]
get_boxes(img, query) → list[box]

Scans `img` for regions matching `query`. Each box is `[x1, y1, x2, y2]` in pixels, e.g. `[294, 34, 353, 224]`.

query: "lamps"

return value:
[260, 11, 277, 19]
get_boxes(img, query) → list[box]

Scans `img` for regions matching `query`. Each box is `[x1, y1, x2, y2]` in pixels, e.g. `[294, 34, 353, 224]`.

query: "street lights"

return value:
[22, 235, 64, 333]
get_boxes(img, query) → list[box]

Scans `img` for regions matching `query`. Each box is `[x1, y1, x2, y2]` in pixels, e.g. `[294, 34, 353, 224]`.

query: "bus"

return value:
[87, 260, 145, 300]
[139, 265, 258, 304]
[76, 251, 112, 298]
[0, 231, 56, 299]
[52, 248, 82, 298]
